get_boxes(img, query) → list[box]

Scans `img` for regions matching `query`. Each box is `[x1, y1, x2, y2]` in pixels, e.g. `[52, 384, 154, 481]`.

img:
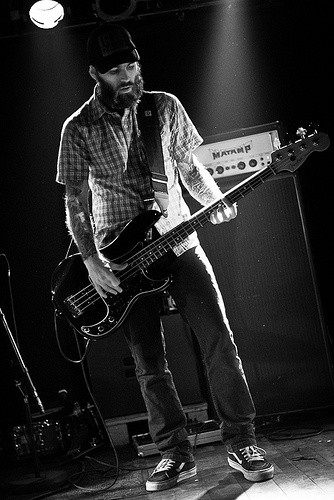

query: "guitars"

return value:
[50, 126, 330, 341]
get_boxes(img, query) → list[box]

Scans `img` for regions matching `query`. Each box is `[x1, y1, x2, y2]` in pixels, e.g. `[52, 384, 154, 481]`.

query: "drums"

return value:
[67, 404, 103, 435]
[31, 406, 72, 436]
[10, 423, 56, 460]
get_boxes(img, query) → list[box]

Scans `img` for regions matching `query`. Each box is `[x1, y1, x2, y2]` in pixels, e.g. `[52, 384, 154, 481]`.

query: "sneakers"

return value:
[227, 445, 274, 482]
[146, 459, 197, 492]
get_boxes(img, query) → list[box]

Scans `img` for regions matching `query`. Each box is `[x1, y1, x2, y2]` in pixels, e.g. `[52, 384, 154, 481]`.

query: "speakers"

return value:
[85, 313, 208, 427]
[179, 172, 334, 421]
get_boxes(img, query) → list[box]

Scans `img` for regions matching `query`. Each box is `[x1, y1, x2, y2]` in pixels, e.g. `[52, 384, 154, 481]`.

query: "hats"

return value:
[87, 24, 140, 74]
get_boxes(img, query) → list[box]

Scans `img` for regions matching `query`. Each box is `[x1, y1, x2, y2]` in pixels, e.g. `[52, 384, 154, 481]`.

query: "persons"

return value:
[56, 26, 274, 491]
